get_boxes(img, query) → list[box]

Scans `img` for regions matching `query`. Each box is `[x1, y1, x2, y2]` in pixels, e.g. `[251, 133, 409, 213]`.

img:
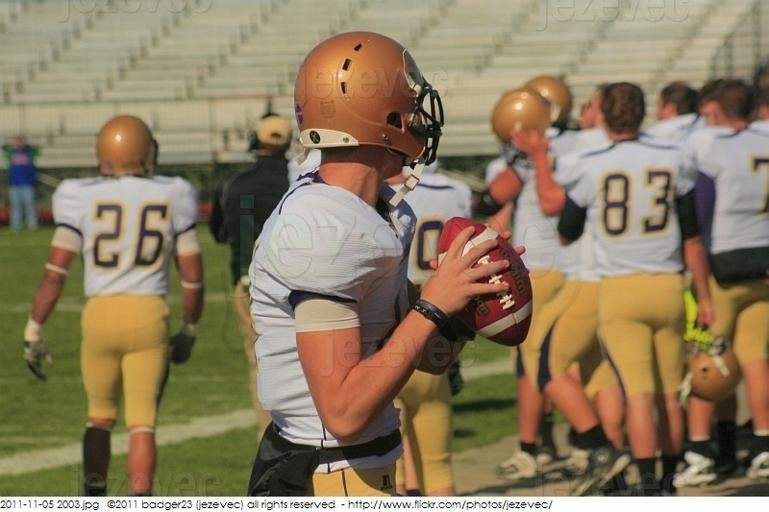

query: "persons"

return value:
[1, 136, 43, 231]
[244, 31, 531, 496]
[474, 68, 769, 497]
[380, 151, 473, 495]
[209, 113, 295, 448]
[22, 115, 206, 496]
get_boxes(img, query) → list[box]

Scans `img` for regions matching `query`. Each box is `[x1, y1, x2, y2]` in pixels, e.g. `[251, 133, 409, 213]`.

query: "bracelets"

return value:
[411, 298, 449, 334]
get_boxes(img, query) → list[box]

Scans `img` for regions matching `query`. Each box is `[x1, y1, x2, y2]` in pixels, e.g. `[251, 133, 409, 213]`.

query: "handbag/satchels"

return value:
[249, 420, 320, 496]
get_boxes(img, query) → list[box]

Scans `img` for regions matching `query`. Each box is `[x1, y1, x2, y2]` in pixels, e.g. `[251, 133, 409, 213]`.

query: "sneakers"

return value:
[744, 435, 769, 479]
[567, 445, 592, 473]
[573, 443, 631, 496]
[494, 450, 537, 478]
[673, 451, 718, 487]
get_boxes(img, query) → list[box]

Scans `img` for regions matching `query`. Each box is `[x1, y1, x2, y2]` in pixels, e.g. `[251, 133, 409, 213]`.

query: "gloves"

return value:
[169, 316, 198, 363]
[24, 319, 52, 380]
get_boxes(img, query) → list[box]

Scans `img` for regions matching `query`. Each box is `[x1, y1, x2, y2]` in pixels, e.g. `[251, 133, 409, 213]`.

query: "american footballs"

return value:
[438, 217, 532, 346]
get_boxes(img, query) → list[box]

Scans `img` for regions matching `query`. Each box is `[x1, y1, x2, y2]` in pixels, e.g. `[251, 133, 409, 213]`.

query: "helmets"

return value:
[523, 76, 571, 121]
[248, 114, 291, 150]
[97, 116, 157, 176]
[687, 337, 740, 402]
[294, 32, 444, 170]
[492, 88, 549, 142]
